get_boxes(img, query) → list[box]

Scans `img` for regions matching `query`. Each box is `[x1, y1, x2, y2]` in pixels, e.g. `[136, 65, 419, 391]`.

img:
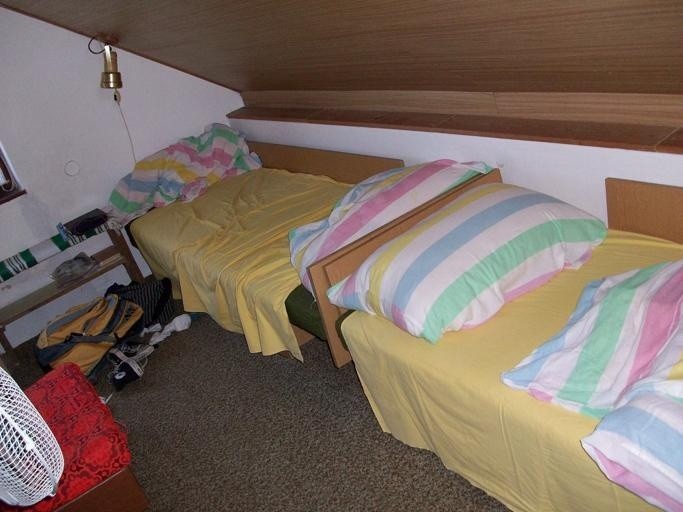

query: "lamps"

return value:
[86, 36, 122, 90]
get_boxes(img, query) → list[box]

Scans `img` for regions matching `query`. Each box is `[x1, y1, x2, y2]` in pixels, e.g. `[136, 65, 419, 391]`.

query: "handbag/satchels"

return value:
[34, 293, 145, 386]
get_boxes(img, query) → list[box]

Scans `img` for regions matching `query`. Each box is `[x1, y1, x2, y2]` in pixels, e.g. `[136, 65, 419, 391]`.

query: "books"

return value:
[91, 245, 122, 267]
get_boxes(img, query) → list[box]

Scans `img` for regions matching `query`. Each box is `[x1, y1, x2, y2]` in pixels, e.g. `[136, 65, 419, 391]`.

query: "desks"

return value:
[15, 361, 149, 510]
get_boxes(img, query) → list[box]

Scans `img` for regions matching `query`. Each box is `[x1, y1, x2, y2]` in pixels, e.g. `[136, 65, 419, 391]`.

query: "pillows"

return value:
[288, 151, 491, 298]
[325, 180, 606, 344]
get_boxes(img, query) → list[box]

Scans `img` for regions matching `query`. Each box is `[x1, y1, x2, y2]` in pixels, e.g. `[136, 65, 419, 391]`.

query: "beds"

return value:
[324, 168, 681, 511]
[125, 140, 404, 371]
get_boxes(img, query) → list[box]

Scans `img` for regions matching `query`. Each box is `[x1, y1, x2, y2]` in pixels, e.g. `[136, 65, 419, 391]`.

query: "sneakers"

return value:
[107, 343, 154, 385]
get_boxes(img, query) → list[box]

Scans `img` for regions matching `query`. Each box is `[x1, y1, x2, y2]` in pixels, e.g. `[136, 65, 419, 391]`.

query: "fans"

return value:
[0, 368, 64, 506]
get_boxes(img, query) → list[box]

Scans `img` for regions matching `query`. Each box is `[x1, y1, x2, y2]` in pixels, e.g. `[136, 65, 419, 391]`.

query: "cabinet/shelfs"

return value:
[0, 216, 145, 368]
